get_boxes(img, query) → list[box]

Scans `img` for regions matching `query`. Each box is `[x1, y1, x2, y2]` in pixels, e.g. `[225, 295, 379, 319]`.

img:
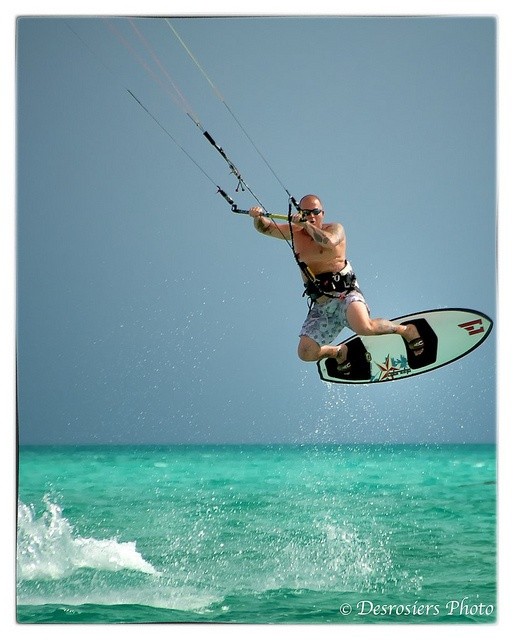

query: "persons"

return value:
[249, 194, 424, 376]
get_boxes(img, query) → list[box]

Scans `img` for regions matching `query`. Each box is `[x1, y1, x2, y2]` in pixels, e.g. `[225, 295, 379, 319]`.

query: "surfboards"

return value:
[317, 309, 494, 385]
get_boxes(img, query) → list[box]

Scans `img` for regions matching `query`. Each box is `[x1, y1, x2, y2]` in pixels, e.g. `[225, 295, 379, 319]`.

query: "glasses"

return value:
[303, 208, 322, 215]
